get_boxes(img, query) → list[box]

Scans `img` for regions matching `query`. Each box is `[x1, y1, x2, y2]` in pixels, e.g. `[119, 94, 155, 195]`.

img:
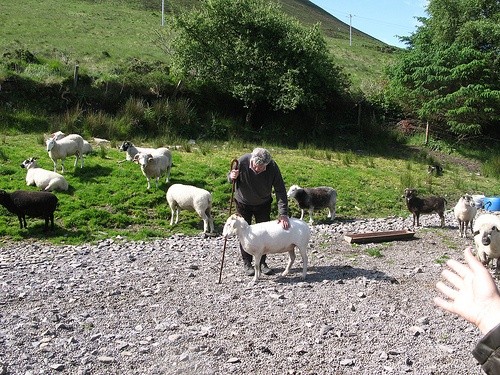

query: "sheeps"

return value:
[452, 191, 480, 238]
[402, 186, 448, 230]
[0, 188, 60, 232]
[19, 155, 69, 193]
[219, 211, 312, 289]
[286, 183, 338, 224]
[45, 130, 92, 174]
[164, 183, 215, 234]
[427, 162, 444, 176]
[118, 140, 172, 190]
[470, 211, 500, 272]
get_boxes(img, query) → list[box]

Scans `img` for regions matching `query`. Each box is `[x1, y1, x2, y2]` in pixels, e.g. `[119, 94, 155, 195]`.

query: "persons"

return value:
[227, 147, 291, 275]
[434, 247, 500, 375]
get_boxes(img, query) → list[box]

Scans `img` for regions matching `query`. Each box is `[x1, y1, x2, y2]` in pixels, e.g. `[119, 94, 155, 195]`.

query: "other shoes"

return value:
[260, 263, 272, 275]
[243, 262, 255, 276]
